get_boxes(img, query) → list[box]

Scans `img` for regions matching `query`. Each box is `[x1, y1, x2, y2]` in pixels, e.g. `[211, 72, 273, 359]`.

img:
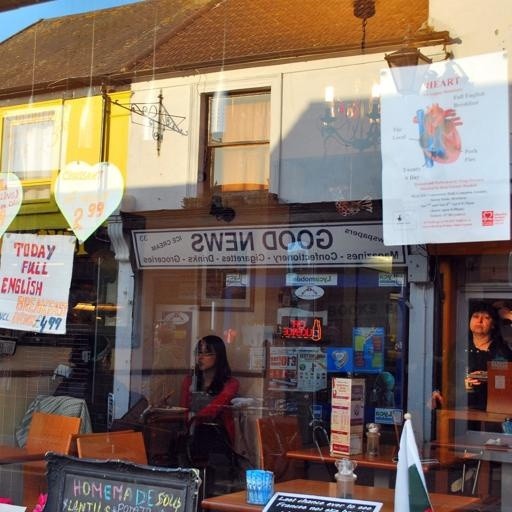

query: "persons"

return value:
[53, 328, 149, 436]
[179, 334, 240, 463]
[457, 301, 512, 434]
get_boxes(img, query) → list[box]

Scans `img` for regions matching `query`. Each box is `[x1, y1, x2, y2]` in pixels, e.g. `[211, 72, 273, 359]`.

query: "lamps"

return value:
[317, 0, 383, 154]
[208, 184, 236, 223]
[14, 1, 232, 149]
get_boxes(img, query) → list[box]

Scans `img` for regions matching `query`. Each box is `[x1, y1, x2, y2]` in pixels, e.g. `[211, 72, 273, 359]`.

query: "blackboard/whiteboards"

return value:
[45, 452, 202, 512]
[261, 491, 384, 512]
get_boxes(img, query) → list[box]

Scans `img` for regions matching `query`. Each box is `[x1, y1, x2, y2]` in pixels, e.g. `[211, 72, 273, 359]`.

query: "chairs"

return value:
[17, 413, 153, 510]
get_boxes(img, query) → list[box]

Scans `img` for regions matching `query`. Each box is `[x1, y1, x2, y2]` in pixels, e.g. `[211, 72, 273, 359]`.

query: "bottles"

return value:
[334, 458, 358, 499]
[364, 423, 382, 459]
[312, 319, 321, 341]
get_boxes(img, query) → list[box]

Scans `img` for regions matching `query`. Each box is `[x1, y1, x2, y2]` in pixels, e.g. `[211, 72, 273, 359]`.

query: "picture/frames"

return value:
[195, 266, 256, 313]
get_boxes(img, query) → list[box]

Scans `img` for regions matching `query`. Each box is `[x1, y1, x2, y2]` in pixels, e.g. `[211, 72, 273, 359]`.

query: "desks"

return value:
[201, 393, 512, 510]
